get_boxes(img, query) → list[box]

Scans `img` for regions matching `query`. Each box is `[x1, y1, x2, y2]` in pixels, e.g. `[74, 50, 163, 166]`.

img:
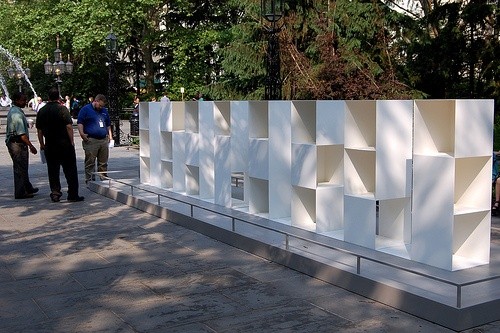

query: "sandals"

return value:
[491, 201, 500, 212]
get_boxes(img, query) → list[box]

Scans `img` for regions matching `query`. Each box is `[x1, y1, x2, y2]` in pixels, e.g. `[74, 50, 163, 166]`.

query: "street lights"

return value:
[8, 45, 31, 92]
[44, 34, 73, 99]
[106, 27, 119, 140]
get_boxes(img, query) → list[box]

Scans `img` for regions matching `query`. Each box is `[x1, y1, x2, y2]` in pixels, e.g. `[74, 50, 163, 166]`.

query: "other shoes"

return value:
[24, 187, 40, 194]
[15, 192, 33, 199]
[70, 196, 84, 202]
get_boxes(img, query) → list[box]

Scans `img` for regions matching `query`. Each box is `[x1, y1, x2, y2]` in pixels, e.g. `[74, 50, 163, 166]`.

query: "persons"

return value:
[77, 94, 113, 188]
[0, 90, 93, 203]
[160, 93, 170, 103]
[151, 97, 156, 102]
[193, 94, 204, 101]
